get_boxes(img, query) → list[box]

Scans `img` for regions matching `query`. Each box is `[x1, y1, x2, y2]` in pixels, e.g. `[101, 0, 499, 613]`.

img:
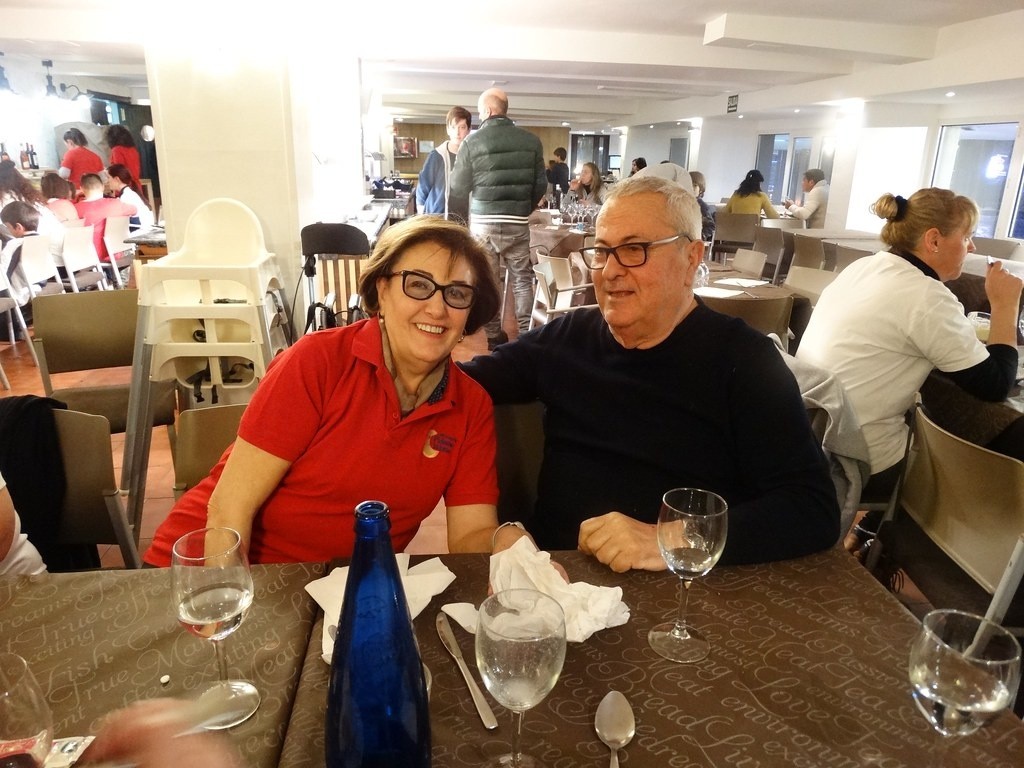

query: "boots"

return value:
[843, 516, 880, 560]
[854, 539, 911, 612]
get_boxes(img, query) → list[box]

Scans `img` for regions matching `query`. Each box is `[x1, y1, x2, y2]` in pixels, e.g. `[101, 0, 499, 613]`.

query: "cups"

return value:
[0, 652, 53, 768]
[967, 311, 991, 346]
[1019, 309, 1024, 336]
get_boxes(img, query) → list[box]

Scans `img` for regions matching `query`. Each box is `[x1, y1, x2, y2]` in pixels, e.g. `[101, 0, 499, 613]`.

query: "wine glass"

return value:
[475, 589, 567, 768]
[909, 610, 1022, 768]
[557, 203, 600, 233]
[692, 242, 712, 289]
[170, 527, 262, 730]
[546, 193, 553, 210]
[647, 488, 729, 663]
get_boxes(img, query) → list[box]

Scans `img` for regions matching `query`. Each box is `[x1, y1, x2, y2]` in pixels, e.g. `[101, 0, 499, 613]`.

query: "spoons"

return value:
[595, 691, 635, 768]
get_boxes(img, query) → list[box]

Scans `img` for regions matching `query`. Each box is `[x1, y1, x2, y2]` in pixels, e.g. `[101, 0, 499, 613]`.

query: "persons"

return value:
[784, 169, 830, 229]
[0, 125, 153, 341]
[722, 169, 780, 222]
[450, 88, 547, 349]
[544, 147, 569, 208]
[0, 472, 49, 574]
[690, 172, 716, 241]
[140, 215, 570, 594]
[628, 157, 647, 177]
[416, 105, 472, 226]
[793, 188, 1024, 587]
[560, 162, 609, 209]
[453, 177, 841, 574]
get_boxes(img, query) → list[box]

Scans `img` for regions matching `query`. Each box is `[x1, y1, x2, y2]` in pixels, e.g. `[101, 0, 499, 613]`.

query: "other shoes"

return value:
[0, 324, 23, 341]
[487, 329, 510, 350]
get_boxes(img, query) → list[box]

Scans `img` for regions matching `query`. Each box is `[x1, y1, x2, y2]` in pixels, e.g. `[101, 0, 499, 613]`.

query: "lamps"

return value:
[0, 51, 94, 113]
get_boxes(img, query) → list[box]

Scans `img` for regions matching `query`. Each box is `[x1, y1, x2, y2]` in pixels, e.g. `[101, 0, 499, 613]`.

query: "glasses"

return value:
[386, 270, 477, 310]
[579, 233, 695, 269]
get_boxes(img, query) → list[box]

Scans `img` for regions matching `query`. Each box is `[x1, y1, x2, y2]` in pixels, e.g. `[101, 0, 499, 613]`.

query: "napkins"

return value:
[544, 225, 560, 231]
[303, 553, 451, 666]
[691, 286, 744, 299]
[567, 229, 588, 235]
[442, 536, 629, 645]
[714, 278, 769, 288]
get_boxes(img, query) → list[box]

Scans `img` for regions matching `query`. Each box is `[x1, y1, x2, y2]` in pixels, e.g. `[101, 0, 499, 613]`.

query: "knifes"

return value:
[436, 612, 499, 729]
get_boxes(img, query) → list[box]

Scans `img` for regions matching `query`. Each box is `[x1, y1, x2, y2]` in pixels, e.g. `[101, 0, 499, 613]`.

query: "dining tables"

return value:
[528, 208, 588, 224]
[689, 261, 812, 355]
[0, 547, 1024, 768]
[785, 227, 1024, 317]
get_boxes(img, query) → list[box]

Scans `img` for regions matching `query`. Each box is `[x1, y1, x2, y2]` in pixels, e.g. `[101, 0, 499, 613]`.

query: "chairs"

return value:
[858, 405, 1024, 655]
[527, 247, 599, 330]
[0, 290, 251, 574]
[968, 236, 1024, 267]
[0, 207, 152, 391]
[702, 213, 874, 301]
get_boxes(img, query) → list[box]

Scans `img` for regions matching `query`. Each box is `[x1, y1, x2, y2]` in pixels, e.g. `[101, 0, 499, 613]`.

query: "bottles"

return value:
[366, 175, 372, 195]
[0, 142, 9, 162]
[20, 143, 31, 169]
[30, 145, 39, 169]
[324, 500, 432, 768]
[555, 184, 560, 190]
[26, 143, 33, 168]
[556, 188, 564, 209]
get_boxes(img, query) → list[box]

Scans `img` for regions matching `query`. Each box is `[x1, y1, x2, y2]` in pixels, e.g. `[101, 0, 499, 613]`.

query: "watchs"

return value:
[492, 521, 524, 547]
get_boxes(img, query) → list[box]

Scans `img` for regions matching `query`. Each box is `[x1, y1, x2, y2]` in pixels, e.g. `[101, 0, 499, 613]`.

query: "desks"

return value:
[529, 223, 597, 265]
